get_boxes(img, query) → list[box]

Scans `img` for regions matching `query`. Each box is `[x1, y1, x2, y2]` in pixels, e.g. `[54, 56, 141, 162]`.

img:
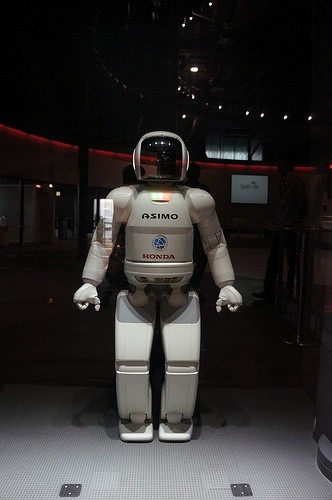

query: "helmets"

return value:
[132, 131, 188, 182]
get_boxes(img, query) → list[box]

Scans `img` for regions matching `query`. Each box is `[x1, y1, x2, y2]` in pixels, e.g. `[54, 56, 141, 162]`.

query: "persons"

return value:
[73, 131, 243, 443]
